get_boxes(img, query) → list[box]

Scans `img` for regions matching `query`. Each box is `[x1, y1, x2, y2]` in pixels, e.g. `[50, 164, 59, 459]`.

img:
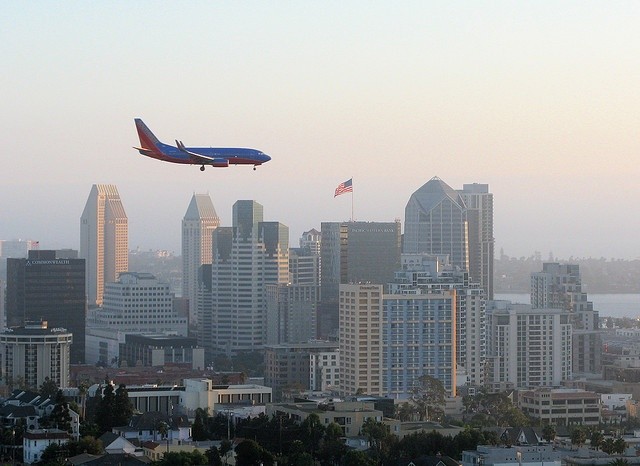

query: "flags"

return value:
[334, 179, 353, 197]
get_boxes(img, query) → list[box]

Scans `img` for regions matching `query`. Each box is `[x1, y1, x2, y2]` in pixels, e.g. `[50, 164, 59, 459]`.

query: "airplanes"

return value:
[132, 118, 271, 171]
